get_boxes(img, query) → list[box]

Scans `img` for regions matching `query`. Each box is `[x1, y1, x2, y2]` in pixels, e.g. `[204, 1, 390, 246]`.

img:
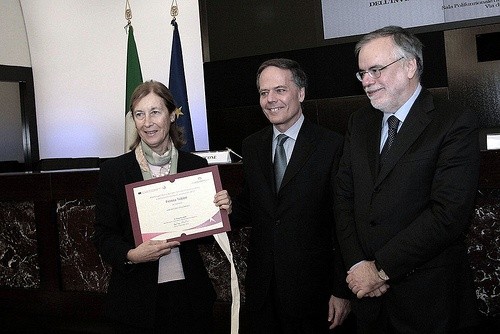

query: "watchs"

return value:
[373, 259, 390, 281]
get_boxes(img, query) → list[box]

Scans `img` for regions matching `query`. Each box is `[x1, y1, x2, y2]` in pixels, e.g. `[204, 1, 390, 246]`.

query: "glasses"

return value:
[356, 55, 409, 82]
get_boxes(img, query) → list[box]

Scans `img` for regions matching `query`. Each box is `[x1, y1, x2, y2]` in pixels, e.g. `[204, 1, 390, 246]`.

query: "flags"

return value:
[169, 18, 196, 153]
[124, 25, 144, 154]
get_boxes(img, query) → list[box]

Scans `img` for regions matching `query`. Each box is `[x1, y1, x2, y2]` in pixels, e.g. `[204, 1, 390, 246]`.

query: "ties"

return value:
[272, 135, 287, 193]
[381, 116, 400, 167]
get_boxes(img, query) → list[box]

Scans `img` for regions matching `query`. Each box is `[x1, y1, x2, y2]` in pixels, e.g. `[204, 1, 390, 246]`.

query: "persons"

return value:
[95, 80, 234, 334]
[222, 57, 353, 334]
[334, 25, 480, 334]
